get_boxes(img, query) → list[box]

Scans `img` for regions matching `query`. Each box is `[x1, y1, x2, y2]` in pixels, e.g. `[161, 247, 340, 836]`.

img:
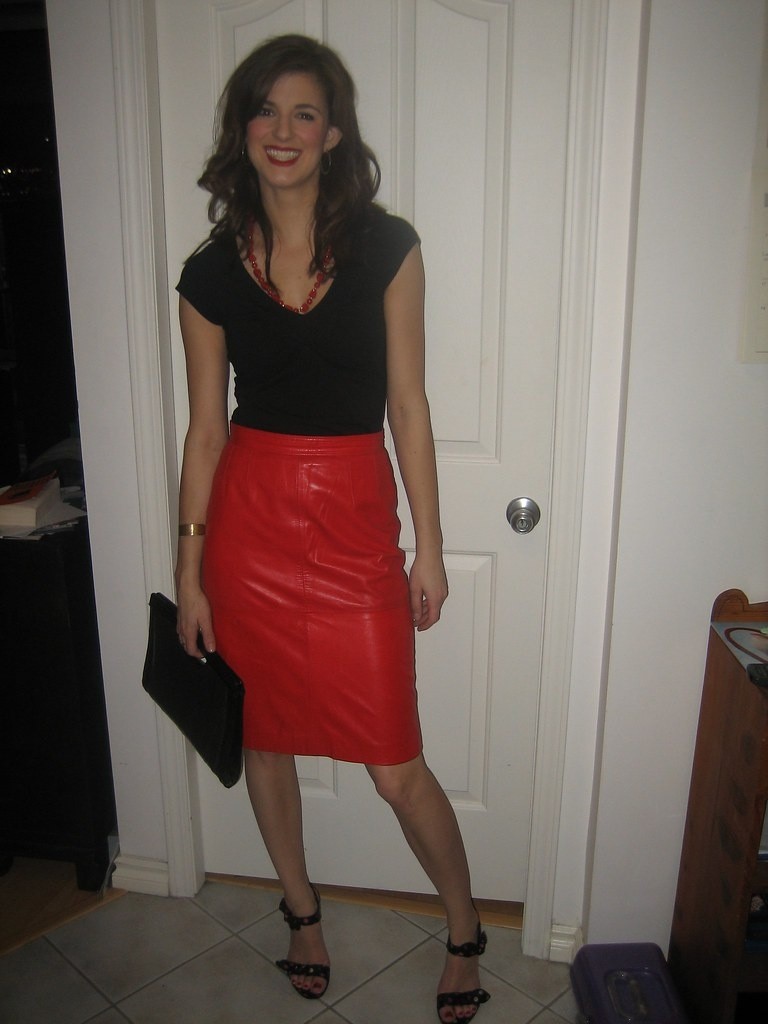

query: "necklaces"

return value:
[235, 217, 338, 312]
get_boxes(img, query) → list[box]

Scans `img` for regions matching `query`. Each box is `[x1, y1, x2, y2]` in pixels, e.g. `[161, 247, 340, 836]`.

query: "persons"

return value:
[174, 33, 493, 1023]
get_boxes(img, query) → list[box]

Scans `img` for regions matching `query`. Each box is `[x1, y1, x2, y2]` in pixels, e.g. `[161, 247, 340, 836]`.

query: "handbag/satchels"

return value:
[142, 591, 246, 789]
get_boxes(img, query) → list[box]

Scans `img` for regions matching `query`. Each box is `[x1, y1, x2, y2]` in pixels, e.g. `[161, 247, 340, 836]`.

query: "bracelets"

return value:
[180, 525, 207, 535]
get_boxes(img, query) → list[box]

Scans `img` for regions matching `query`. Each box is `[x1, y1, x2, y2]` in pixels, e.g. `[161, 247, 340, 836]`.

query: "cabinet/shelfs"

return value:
[666, 588, 768, 1024]
[0, 521, 116, 895]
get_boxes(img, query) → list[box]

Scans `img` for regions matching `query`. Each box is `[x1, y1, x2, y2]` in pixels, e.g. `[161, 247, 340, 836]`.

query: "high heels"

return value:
[275, 882, 332, 1000]
[436, 920, 490, 1024]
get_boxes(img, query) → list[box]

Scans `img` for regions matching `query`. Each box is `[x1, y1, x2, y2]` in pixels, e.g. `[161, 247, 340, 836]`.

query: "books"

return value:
[0, 467, 63, 531]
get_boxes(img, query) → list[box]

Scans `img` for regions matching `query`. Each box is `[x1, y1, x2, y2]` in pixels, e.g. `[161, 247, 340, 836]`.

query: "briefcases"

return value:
[570, 942, 688, 1024]
